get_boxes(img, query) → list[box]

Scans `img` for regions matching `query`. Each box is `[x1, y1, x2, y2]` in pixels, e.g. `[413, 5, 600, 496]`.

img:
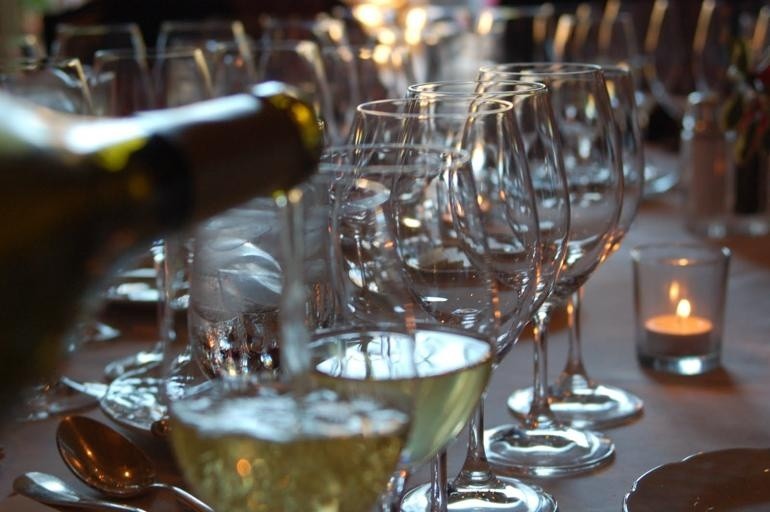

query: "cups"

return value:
[627, 240, 730, 378]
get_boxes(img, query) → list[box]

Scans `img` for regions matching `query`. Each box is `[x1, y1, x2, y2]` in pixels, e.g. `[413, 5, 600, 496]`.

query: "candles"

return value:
[646, 299, 713, 356]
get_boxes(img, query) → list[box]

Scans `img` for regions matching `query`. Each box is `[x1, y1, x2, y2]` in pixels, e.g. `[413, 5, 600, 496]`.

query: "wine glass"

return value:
[647, 0, 770, 237]
[1, 0, 648, 512]
